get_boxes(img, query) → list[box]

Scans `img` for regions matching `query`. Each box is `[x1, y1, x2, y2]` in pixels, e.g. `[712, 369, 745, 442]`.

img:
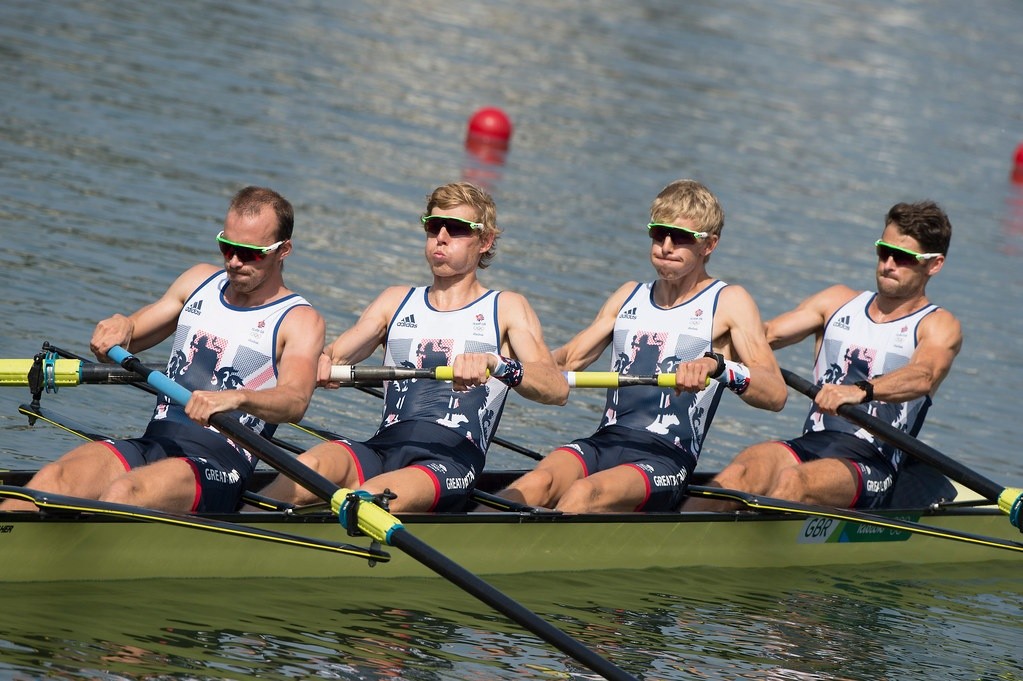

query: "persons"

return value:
[0, 186, 325, 516]
[681, 201, 963, 512]
[238, 183, 568, 513]
[474, 180, 790, 511]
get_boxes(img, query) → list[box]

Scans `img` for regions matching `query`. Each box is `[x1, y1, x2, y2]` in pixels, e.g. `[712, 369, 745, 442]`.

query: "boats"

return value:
[0, 468, 1023, 584]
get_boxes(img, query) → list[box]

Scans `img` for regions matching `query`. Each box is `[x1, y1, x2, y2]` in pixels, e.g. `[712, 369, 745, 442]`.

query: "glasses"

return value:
[421, 215, 486, 236]
[874, 239, 943, 265]
[647, 222, 709, 244]
[215, 231, 287, 262]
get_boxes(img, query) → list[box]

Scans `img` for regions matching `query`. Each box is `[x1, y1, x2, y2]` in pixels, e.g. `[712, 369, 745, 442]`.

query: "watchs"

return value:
[703, 351, 726, 378]
[855, 379, 875, 404]
